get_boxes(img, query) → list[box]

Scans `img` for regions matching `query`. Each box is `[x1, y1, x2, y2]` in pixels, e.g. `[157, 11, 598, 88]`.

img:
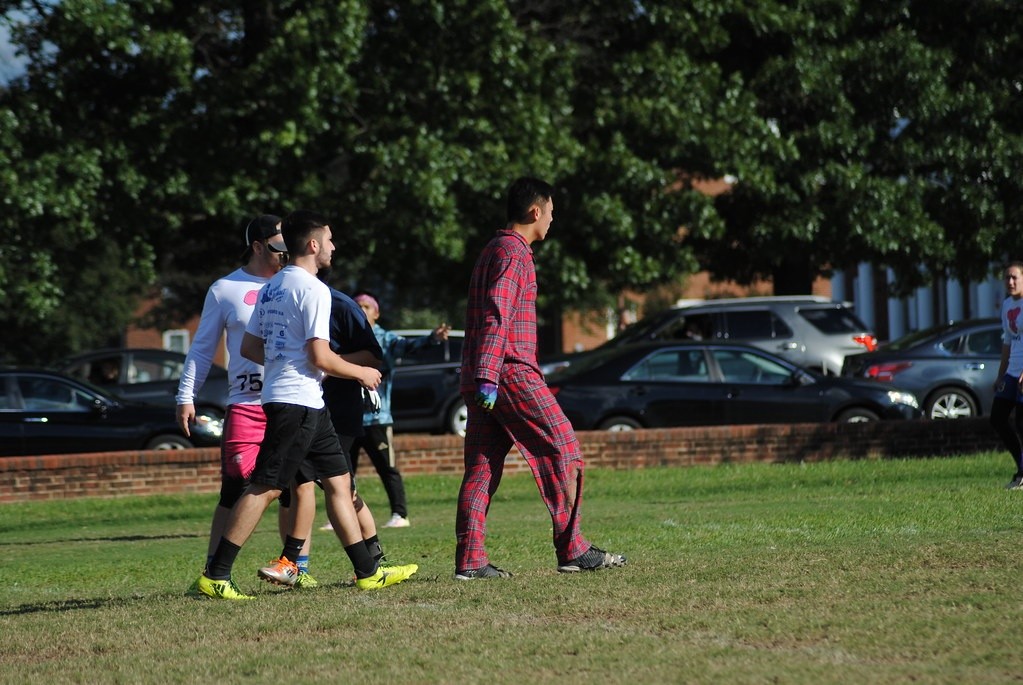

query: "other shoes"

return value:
[1006, 474, 1023, 490]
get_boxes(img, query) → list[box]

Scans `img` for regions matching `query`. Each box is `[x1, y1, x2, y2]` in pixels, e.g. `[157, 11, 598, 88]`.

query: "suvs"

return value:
[539, 296, 878, 376]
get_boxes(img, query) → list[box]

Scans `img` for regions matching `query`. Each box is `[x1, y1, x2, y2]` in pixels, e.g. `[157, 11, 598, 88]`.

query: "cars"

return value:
[840, 317, 1004, 419]
[542, 341, 922, 431]
[385, 330, 467, 436]
[42, 348, 227, 405]
[0, 367, 225, 451]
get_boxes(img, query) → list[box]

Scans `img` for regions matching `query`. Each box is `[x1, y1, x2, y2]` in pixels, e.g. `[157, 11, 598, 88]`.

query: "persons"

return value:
[454, 177, 626, 581]
[196, 211, 418, 600]
[319, 295, 451, 531]
[989, 260, 1023, 490]
[685, 319, 703, 340]
[257, 261, 390, 586]
[175, 214, 318, 588]
[100, 361, 119, 386]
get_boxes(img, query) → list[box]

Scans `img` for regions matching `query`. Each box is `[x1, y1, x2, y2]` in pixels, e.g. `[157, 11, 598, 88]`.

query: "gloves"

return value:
[475, 380, 498, 411]
[365, 385, 381, 414]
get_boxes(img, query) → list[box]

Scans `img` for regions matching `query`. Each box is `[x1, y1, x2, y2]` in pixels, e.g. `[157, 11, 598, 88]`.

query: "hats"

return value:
[239, 214, 289, 263]
[266, 241, 287, 253]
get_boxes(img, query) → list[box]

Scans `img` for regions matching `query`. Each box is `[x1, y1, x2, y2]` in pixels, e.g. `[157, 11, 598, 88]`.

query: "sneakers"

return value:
[382, 513, 410, 528]
[258, 556, 298, 588]
[455, 563, 514, 580]
[357, 559, 419, 592]
[195, 573, 257, 600]
[558, 544, 627, 572]
[295, 571, 318, 589]
